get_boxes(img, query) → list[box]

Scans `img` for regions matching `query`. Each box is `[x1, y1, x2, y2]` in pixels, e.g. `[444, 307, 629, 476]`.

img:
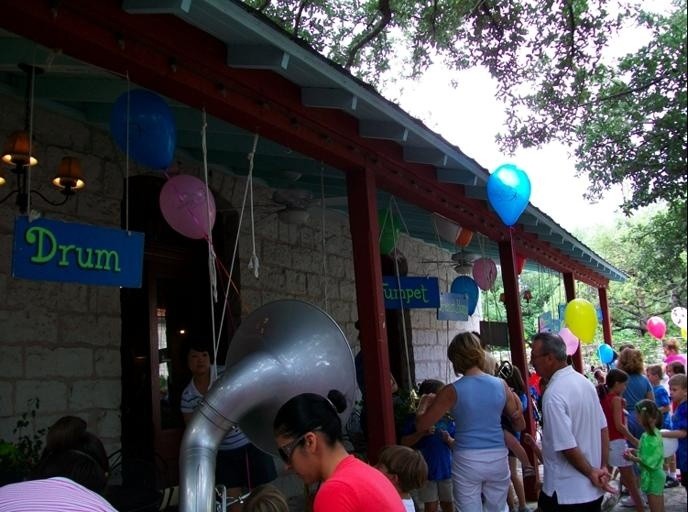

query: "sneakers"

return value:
[665, 477, 679, 488]
[621, 496, 646, 507]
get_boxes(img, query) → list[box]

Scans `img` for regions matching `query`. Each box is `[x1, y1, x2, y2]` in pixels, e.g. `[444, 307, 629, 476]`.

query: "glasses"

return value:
[277, 426, 323, 465]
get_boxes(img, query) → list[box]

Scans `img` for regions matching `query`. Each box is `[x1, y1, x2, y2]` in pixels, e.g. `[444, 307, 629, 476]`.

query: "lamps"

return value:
[522, 290, 533, 304]
[0, 62, 88, 215]
[276, 206, 310, 225]
[454, 263, 473, 274]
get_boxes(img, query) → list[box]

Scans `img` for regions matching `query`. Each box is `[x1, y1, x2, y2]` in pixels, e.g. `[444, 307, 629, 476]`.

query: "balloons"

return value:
[597, 342, 616, 363]
[450, 276, 479, 317]
[377, 210, 399, 256]
[107, 86, 176, 170]
[455, 229, 473, 249]
[645, 316, 666, 340]
[671, 306, 687, 329]
[473, 258, 499, 287]
[565, 297, 595, 342]
[659, 428, 679, 459]
[517, 254, 526, 276]
[559, 328, 578, 357]
[158, 174, 215, 237]
[486, 162, 531, 228]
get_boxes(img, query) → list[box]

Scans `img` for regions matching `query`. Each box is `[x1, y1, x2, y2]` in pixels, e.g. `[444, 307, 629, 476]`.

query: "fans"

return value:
[417, 245, 475, 264]
[216, 169, 348, 231]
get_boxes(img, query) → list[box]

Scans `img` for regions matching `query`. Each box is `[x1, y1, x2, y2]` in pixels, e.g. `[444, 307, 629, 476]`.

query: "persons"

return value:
[415, 331, 526, 512]
[669, 374, 688, 490]
[646, 365, 679, 488]
[272, 388, 406, 512]
[179, 338, 252, 489]
[373, 446, 427, 512]
[664, 342, 684, 366]
[528, 329, 618, 511]
[622, 399, 667, 512]
[501, 364, 534, 512]
[45, 415, 87, 446]
[617, 346, 654, 508]
[667, 362, 685, 376]
[595, 368, 647, 512]
[400, 379, 455, 512]
[0, 446, 120, 512]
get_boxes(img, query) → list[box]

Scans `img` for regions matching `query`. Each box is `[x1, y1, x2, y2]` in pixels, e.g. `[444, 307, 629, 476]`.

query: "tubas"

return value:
[181, 297, 358, 512]
